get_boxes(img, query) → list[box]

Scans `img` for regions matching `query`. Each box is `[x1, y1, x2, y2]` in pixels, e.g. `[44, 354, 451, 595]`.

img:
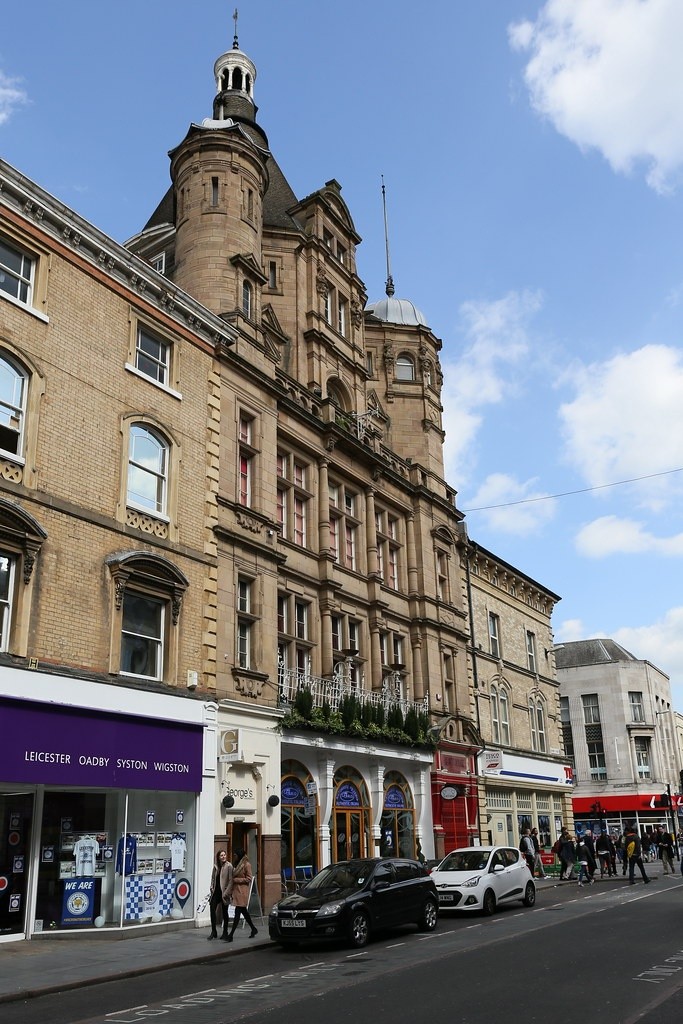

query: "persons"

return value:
[583, 829, 597, 870]
[626, 828, 651, 885]
[207, 849, 233, 940]
[224, 848, 258, 942]
[575, 837, 595, 887]
[557, 827, 683, 887]
[520, 827, 535, 878]
[654, 826, 675, 875]
[596, 831, 613, 878]
[530, 828, 551, 880]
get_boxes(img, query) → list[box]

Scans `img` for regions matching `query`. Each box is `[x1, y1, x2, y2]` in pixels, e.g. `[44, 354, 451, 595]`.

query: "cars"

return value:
[268, 856, 441, 948]
[428, 844, 536, 916]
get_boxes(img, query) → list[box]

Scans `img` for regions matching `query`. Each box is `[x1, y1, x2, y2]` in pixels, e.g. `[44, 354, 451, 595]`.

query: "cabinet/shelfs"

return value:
[60, 831, 109, 878]
[121, 832, 186, 873]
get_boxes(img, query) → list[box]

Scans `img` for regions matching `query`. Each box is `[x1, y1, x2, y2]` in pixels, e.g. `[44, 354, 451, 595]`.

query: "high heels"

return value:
[220, 931, 228, 939]
[207, 931, 217, 940]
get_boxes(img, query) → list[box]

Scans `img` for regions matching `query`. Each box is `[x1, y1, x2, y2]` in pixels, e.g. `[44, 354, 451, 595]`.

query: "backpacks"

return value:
[626, 841, 636, 858]
[519, 836, 531, 852]
[551, 840, 560, 853]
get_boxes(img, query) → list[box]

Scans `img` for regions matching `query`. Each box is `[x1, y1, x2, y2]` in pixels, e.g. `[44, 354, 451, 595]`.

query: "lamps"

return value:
[332, 648, 360, 684]
[656, 710, 669, 716]
[382, 663, 406, 696]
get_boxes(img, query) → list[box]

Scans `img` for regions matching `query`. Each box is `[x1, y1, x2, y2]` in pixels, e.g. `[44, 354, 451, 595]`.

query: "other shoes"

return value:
[533, 877, 540, 881]
[663, 872, 668, 875]
[226, 935, 233, 942]
[560, 876, 570, 880]
[645, 880, 651, 884]
[578, 881, 584, 887]
[589, 879, 594, 886]
[601, 874, 618, 879]
[630, 882, 636, 885]
[570, 877, 577, 880]
[248, 927, 258, 938]
[672, 870, 675, 873]
[544, 875, 552, 880]
[623, 870, 626, 875]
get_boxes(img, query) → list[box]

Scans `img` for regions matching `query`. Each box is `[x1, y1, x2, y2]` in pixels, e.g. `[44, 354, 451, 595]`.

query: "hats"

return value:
[233, 844, 246, 857]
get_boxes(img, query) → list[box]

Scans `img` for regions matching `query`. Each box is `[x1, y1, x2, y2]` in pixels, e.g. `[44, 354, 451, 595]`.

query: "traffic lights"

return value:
[660, 794, 668, 807]
[602, 808, 607, 818]
[590, 804, 597, 819]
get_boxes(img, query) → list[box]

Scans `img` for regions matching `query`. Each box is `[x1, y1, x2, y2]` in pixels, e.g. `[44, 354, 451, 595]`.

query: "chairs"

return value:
[467, 852, 512, 868]
[281, 865, 317, 898]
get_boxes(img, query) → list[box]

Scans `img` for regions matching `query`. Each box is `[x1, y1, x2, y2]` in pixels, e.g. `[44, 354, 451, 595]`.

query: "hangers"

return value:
[126, 832, 130, 838]
[85, 833, 91, 839]
[176, 832, 181, 838]
